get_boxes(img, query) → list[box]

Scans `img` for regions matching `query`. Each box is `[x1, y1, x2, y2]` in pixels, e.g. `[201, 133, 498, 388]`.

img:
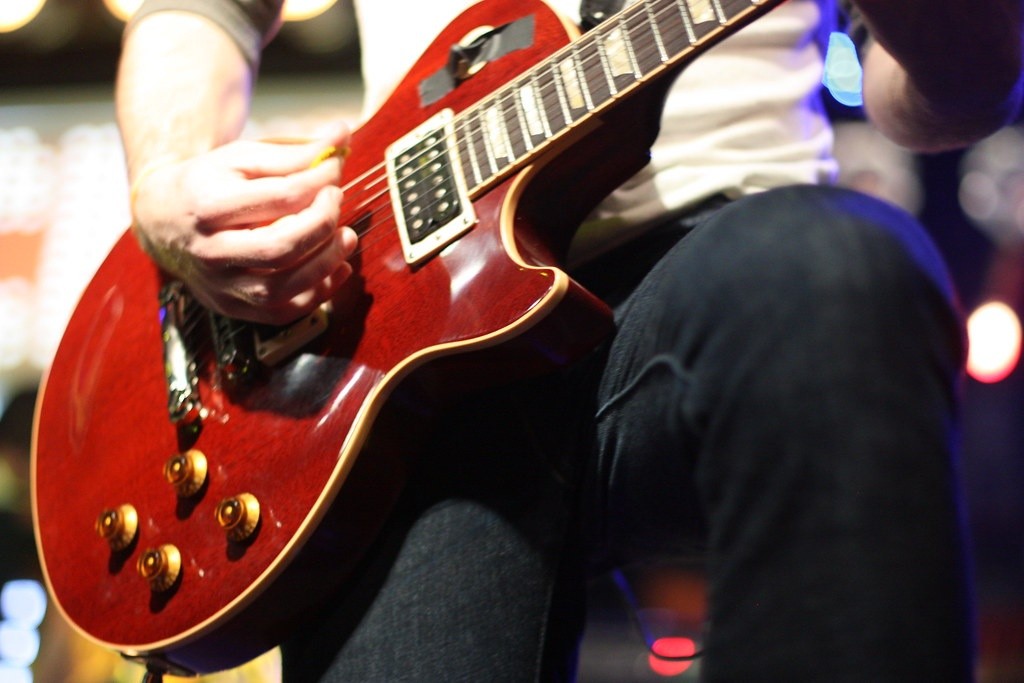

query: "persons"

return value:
[819, 51, 1022, 612]
[114, 0, 1022, 683]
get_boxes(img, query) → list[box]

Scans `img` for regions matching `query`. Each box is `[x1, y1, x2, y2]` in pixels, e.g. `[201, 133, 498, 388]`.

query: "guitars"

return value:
[29, 0, 778, 678]
